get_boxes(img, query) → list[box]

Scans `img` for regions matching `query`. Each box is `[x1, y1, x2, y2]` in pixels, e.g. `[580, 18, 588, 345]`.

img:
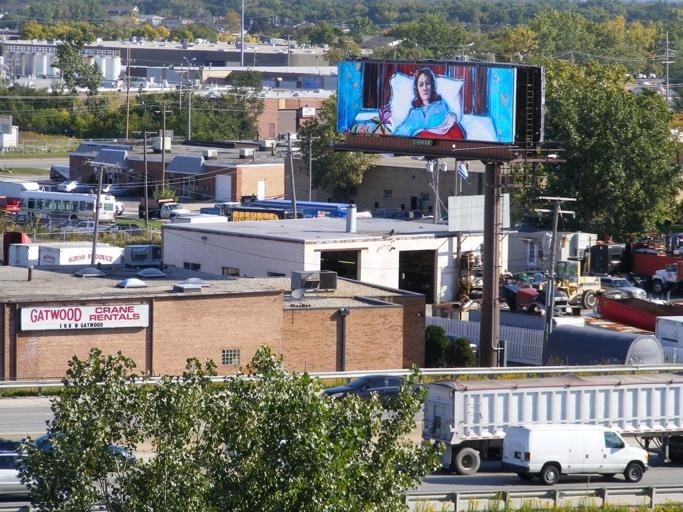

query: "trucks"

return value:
[8, 242, 109, 265]
[651, 261, 683, 294]
[38, 245, 125, 266]
[125, 244, 161, 271]
[501, 424, 650, 484]
[229, 207, 305, 222]
[139, 197, 191, 219]
[201, 202, 242, 220]
[421, 372, 683, 475]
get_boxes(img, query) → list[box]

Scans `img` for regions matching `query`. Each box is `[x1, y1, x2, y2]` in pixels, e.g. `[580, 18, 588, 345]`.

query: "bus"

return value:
[247, 200, 354, 220]
[20, 190, 117, 224]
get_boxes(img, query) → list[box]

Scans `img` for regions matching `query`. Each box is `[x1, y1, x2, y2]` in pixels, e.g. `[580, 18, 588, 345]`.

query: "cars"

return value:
[322, 374, 423, 411]
[97, 183, 129, 197]
[30, 433, 137, 472]
[0, 451, 69, 501]
[4, 212, 145, 237]
[58, 181, 98, 193]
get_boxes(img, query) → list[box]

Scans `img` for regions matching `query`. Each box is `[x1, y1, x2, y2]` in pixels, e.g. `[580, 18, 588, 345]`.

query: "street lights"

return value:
[251, 46, 258, 67]
[183, 56, 197, 87]
[188, 91, 193, 141]
[426, 159, 448, 223]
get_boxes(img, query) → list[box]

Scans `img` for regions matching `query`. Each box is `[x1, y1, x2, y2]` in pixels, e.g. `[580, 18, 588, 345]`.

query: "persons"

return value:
[390, 68, 466, 140]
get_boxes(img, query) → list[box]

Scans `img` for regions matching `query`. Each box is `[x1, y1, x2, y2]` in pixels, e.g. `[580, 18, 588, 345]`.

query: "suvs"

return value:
[599, 277, 648, 301]
[176, 79, 200, 91]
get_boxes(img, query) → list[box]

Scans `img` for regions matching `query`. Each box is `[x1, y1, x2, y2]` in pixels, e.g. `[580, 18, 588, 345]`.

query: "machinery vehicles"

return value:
[538, 259, 602, 310]
